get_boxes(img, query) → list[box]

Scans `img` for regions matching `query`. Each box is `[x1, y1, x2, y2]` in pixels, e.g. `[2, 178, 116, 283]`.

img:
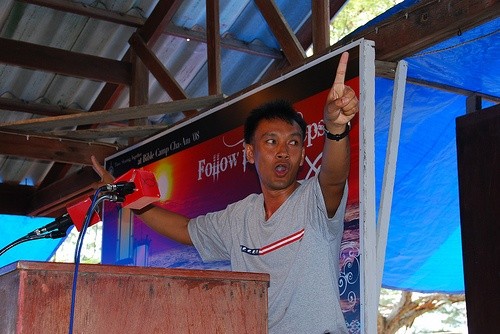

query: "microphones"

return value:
[28, 193, 103, 239]
[98, 168, 161, 211]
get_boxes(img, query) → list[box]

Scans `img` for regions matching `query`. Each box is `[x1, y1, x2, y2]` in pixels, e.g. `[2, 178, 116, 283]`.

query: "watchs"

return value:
[321, 119, 351, 140]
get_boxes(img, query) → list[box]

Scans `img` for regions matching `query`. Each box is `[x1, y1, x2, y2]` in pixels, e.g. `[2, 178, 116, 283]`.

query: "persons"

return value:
[90, 51, 360, 334]
